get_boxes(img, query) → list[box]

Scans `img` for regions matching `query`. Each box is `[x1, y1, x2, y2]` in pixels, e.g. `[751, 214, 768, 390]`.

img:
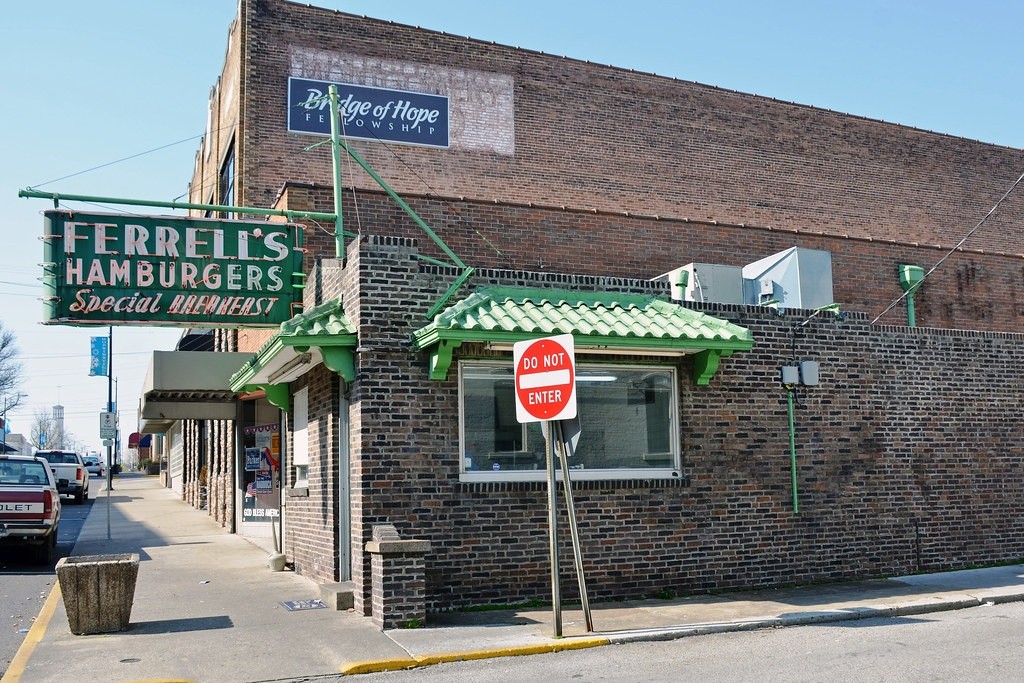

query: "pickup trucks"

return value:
[18, 449, 92, 502]
[0, 455, 62, 570]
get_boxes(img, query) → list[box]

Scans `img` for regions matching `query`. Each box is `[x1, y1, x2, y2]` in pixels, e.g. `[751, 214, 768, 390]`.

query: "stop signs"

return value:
[513, 334, 578, 423]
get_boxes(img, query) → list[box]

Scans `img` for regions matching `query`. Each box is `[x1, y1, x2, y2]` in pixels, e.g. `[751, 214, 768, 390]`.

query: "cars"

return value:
[82, 455, 103, 477]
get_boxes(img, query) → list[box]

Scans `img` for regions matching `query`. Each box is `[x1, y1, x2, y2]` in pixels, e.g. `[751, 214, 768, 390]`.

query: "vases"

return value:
[54, 549, 143, 636]
